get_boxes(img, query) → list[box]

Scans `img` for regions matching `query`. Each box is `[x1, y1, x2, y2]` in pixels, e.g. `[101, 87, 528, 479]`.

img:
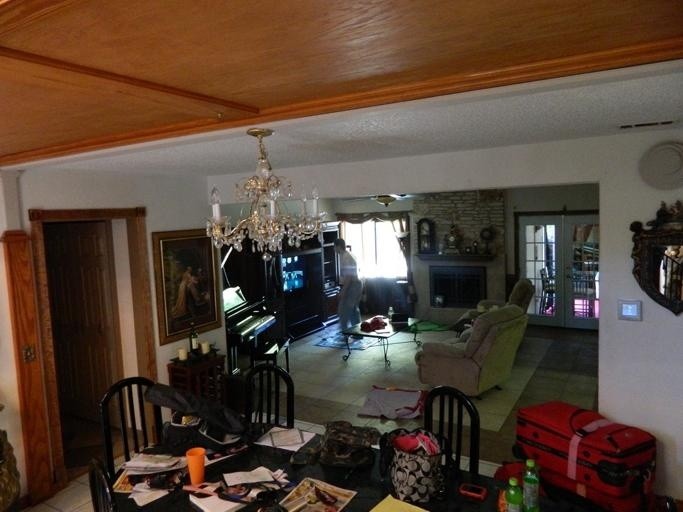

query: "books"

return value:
[126, 454, 181, 467]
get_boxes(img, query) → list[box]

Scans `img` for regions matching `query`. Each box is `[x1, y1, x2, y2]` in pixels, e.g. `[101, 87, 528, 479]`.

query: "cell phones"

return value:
[459, 483, 487, 499]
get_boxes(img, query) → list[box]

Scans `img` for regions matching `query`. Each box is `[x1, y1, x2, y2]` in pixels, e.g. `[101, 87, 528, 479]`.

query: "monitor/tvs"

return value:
[281, 255, 306, 297]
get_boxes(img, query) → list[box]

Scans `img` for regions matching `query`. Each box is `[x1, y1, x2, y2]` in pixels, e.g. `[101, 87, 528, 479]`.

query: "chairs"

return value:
[247, 363, 295, 429]
[415, 303, 532, 399]
[539, 269, 597, 318]
[424, 383, 482, 474]
[84, 456, 118, 510]
[468, 278, 536, 317]
[98, 378, 165, 477]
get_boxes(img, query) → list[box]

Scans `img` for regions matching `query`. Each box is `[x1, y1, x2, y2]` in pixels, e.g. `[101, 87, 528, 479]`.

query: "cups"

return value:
[186, 447, 206, 485]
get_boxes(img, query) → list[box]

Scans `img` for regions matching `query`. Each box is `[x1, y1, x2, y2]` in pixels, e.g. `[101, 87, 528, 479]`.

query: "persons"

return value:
[334, 238, 363, 339]
[171, 266, 198, 319]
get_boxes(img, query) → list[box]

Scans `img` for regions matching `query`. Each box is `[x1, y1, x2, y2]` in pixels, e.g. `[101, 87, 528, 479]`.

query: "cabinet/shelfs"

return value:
[220, 220, 342, 346]
[167, 348, 227, 423]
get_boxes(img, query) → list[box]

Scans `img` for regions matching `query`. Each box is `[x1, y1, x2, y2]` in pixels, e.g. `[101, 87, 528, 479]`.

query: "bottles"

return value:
[505, 477, 523, 512]
[522, 459, 541, 512]
[189, 322, 199, 358]
[388, 305, 394, 321]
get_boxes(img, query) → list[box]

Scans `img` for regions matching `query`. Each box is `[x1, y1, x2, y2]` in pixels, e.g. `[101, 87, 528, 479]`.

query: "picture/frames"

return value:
[151, 227, 223, 346]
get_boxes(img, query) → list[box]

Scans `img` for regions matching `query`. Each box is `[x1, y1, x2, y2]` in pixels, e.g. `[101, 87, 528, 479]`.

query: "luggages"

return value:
[514, 401, 656, 511]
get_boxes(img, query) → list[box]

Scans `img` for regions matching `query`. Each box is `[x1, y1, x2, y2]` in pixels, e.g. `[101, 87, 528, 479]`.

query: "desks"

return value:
[94, 416, 597, 511]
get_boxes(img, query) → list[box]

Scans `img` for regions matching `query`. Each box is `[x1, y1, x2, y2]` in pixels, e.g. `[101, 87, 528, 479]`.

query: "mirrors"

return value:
[629, 199, 683, 316]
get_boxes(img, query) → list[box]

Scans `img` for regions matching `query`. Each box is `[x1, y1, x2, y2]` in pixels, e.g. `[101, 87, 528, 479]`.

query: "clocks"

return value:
[416, 217, 437, 254]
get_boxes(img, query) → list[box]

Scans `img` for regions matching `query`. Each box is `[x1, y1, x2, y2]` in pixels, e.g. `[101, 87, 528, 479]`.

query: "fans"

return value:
[343, 193, 406, 207]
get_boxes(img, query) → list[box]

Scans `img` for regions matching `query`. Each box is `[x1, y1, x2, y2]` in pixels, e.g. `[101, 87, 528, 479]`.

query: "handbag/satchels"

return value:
[160, 409, 245, 452]
[319, 420, 383, 465]
[0, 427, 22, 511]
[382, 425, 449, 505]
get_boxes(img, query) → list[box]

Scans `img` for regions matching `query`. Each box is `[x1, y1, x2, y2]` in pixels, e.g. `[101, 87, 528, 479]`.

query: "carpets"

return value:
[240, 313, 553, 433]
[314, 318, 383, 351]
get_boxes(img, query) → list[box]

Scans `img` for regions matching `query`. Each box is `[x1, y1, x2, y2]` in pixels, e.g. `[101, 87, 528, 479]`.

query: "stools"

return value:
[249, 336, 291, 390]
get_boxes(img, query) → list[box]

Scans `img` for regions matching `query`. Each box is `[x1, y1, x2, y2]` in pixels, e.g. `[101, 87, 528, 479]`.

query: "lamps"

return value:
[202, 128, 327, 263]
